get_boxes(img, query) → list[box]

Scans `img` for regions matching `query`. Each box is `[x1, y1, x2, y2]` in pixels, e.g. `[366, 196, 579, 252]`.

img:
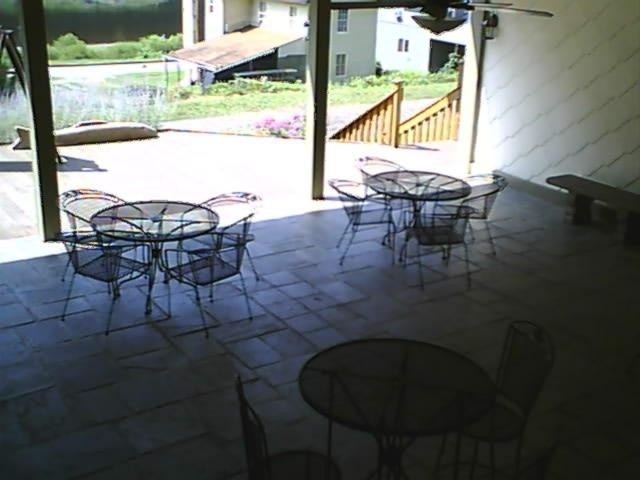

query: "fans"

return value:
[330, 0, 554, 35]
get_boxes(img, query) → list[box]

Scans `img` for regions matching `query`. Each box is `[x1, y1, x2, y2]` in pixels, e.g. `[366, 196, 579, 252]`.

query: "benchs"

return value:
[546, 174, 640, 245]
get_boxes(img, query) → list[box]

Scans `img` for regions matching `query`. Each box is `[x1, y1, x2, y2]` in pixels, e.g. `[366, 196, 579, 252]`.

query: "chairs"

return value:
[432, 319, 556, 480]
[398, 189, 476, 292]
[327, 178, 398, 268]
[55, 230, 154, 336]
[160, 211, 254, 336]
[432, 174, 507, 260]
[179, 190, 265, 298]
[236, 372, 343, 480]
[54, 187, 145, 295]
[353, 155, 420, 243]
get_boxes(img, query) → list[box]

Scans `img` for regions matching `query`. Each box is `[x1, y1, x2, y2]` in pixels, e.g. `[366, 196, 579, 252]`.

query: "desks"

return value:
[298, 336, 497, 480]
[368, 169, 471, 260]
[89, 201, 218, 316]
